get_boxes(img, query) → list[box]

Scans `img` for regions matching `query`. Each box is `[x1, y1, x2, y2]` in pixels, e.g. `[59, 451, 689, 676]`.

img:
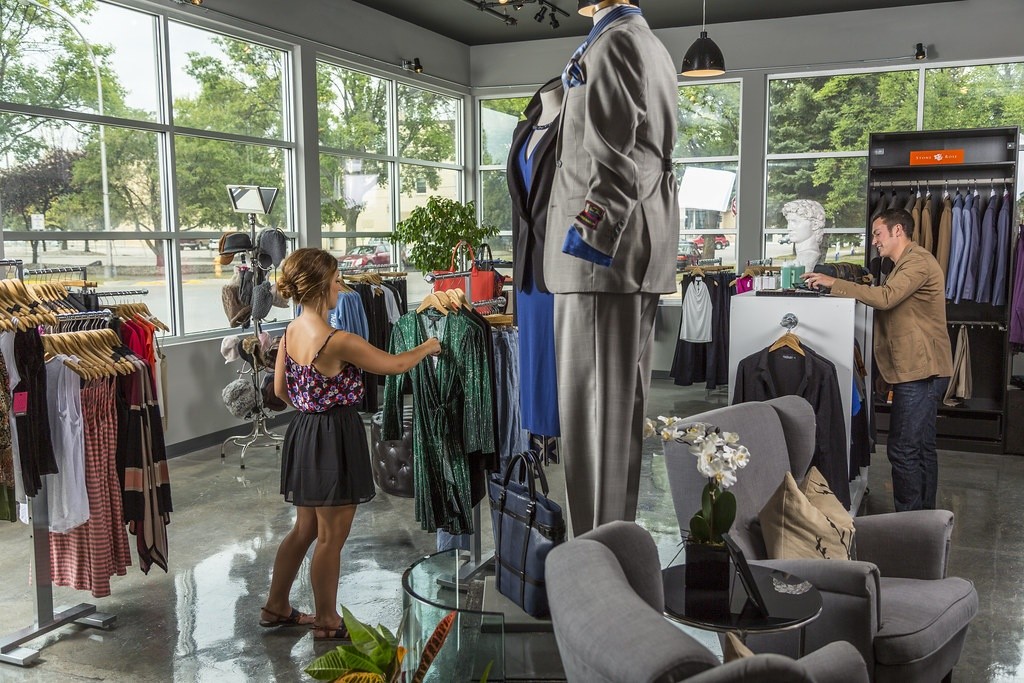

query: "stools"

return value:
[371, 406, 416, 498]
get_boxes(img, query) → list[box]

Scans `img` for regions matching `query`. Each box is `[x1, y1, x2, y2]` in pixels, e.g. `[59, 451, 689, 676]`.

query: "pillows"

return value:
[761, 465, 856, 564]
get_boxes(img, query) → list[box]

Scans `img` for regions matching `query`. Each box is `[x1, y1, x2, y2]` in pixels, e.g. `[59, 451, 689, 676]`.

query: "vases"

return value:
[686, 543, 730, 589]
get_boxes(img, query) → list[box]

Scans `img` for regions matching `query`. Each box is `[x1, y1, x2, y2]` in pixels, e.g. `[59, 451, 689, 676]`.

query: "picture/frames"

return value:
[723, 533, 770, 619]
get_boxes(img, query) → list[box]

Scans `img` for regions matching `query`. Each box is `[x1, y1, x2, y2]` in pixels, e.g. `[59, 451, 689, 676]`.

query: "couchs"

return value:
[663, 396, 977, 683]
[544, 520, 870, 683]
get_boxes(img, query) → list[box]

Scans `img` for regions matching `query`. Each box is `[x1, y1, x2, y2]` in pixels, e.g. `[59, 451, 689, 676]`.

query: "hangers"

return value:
[685, 263, 734, 286]
[871, 177, 1024, 239]
[772, 322, 805, 356]
[332, 261, 514, 328]
[0, 259, 171, 381]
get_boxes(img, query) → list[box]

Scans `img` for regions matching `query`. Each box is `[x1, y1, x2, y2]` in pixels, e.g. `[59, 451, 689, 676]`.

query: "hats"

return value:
[218, 228, 292, 420]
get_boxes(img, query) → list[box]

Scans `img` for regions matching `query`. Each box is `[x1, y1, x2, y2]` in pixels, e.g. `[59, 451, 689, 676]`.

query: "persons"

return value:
[798, 206, 954, 514]
[782, 199, 826, 280]
[505, 1, 682, 540]
[259, 247, 443, 640]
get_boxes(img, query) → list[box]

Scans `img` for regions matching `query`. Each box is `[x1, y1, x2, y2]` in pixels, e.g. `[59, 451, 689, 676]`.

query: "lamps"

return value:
[915, 43, 924, 59]
[498, 0, 559, 29]
[402, 58, 423, 74]
[681, 0, 725, 76]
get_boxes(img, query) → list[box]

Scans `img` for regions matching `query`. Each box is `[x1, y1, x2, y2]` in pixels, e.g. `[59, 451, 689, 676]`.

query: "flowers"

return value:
[643, 414, 751, 543]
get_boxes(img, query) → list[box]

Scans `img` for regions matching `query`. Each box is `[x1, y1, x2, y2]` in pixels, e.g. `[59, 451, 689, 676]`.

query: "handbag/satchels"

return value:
[488, 449, 566, 618]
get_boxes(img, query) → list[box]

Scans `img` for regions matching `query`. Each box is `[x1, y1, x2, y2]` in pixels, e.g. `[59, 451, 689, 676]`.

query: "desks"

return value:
[481, 576, 567, 682]
[659, 563, 822, 658]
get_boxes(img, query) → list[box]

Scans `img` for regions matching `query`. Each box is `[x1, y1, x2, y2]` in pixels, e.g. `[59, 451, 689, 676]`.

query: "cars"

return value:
[179, 239, 211, 250]
[676, 244, 703, 271]
[679, 241, 698, 249]
[778, 236, 792, 244]
[208, 238, 220, 251]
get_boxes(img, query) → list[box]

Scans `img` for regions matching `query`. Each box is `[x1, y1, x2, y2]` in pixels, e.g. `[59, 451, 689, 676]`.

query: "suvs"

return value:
[691, 234, 729, 250]
[336, 244, 407, 275]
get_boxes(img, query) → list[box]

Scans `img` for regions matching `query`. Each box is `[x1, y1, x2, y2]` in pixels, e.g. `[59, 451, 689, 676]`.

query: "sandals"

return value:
[258, 606, 352, 640]
